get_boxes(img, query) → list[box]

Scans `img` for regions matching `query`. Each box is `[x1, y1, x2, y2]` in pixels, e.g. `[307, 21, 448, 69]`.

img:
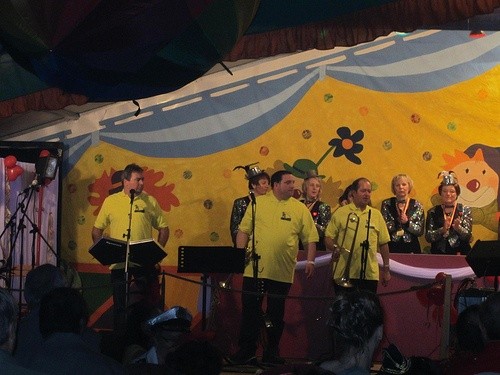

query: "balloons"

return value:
[4, 155, 24, 181]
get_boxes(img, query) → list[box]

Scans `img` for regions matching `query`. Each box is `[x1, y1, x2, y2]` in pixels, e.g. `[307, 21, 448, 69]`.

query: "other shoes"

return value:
[226, 352, 257, 366]
[262, 353, 285, 364]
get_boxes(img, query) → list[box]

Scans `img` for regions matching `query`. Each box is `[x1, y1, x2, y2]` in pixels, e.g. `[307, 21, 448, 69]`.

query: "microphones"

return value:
[19, 180, 38, 195]
[249, 191, 257, 204]
[130, 188, 135, 203]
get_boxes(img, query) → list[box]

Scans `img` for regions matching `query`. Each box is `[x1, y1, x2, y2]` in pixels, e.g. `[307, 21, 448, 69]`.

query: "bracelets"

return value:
[307, 261, 316, 266]
[383, 265, 389, 268]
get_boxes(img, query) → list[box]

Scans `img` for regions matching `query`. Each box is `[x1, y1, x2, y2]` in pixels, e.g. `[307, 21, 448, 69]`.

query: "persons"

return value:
[0, 264, 225, 375]
[324, 177, 391, 297]
[230, 166, 473, 255]
[91, 164, 170, 347]
[307, 292, 500, 375]
[225, 170, 320, 365]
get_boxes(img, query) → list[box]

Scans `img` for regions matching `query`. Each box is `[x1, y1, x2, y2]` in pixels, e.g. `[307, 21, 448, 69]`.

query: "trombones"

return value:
[310, 212, 360, 321]
